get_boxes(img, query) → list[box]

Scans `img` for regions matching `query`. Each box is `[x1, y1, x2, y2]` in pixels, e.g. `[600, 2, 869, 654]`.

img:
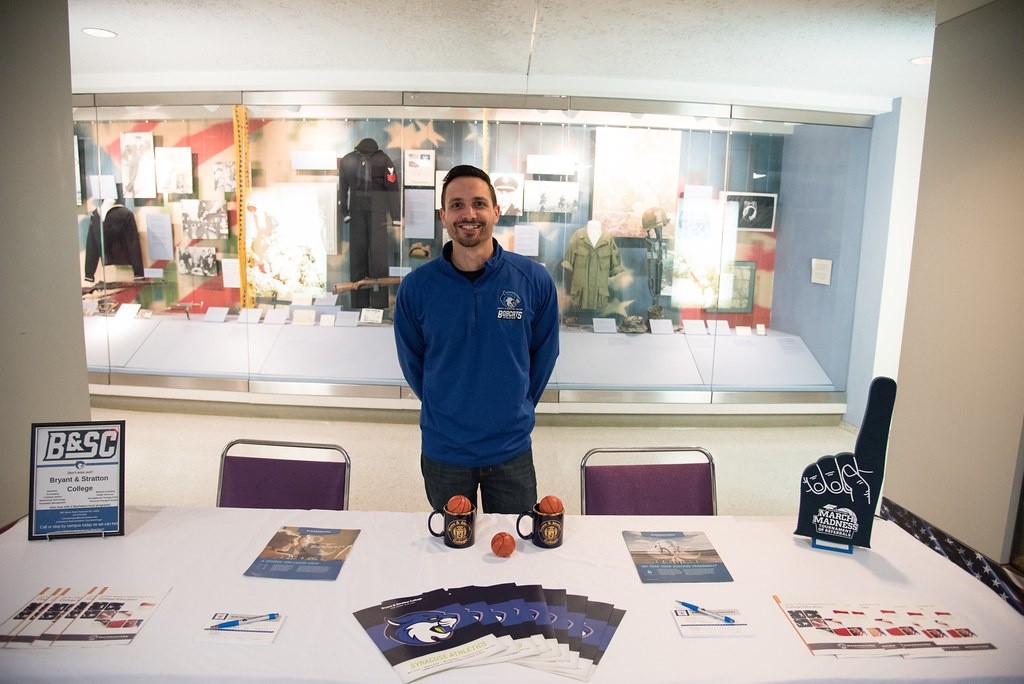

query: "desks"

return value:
[0, 506, 1024, 684]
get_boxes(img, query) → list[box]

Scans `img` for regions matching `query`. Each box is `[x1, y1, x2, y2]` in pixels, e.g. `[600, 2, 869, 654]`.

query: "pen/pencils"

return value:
[211, 612, 279, 631]
[675, 598, 736, 625]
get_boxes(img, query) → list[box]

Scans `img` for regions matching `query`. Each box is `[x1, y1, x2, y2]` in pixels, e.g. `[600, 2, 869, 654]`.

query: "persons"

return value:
[84, 199, 146, 284]
[393, 165, 560, 516]
[182, 247, 218, 277]
[494, 175, 522, 216]
[247, 201, 281, 277]
[338, 139, 400, 309]
[561, 220, 624, 311]
[124, 135, 147, 192]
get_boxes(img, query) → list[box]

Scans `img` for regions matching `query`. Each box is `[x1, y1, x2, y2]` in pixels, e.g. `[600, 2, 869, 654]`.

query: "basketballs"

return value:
[539, 495, 564, 514]
[491, 531, 516, 558]
[447, 495, 472, 514]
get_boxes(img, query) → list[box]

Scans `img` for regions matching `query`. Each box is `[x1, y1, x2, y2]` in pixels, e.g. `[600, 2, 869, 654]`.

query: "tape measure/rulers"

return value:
[232, 103, 259, 309]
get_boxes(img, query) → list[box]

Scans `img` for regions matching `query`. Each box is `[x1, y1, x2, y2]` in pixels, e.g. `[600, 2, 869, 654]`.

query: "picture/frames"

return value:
[27, 419, 126, 540]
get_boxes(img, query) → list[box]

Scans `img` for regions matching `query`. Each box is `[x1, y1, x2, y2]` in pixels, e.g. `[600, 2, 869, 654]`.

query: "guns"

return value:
[652, 225, 664, 310]
[332, 275, 405, 296]
[82, 278, 169, 297]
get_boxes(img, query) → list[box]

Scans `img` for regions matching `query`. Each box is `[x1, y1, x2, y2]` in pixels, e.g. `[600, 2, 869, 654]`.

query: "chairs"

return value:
[216, 439, 351, 511]
[579, 448, 717, 515]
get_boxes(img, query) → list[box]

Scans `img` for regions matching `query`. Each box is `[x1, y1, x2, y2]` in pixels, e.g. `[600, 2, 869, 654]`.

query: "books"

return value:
[353, 579, 627, 682]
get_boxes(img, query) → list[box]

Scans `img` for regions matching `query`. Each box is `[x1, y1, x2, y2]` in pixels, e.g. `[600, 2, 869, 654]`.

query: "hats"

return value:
[642, 207, 670, 228]
